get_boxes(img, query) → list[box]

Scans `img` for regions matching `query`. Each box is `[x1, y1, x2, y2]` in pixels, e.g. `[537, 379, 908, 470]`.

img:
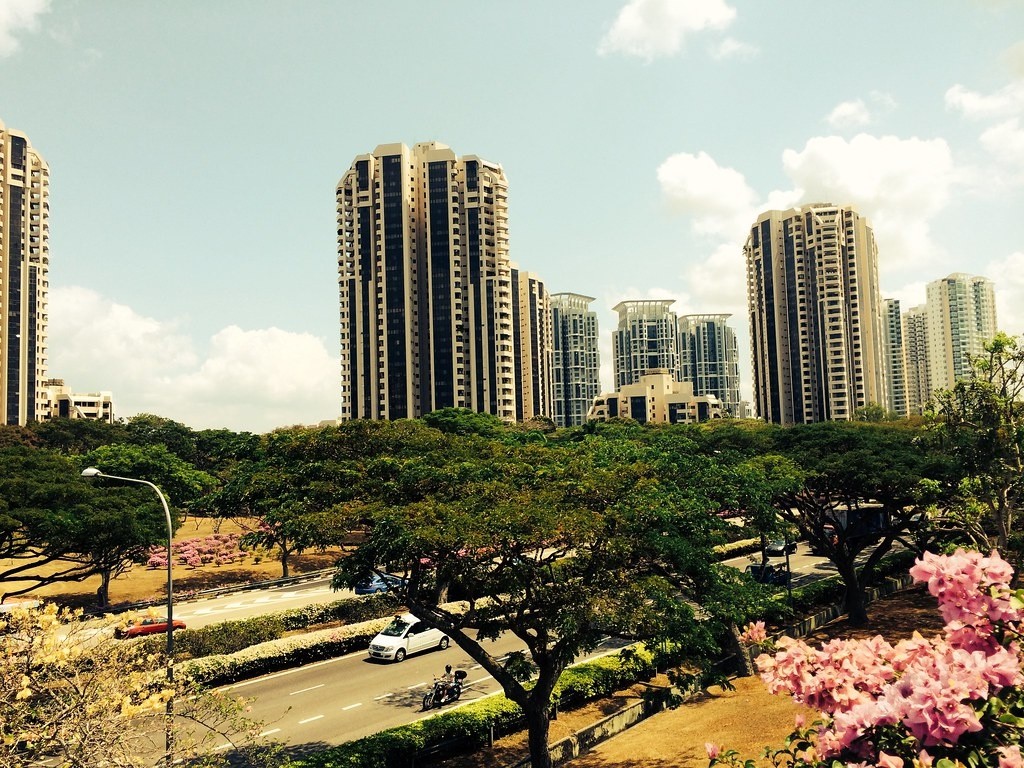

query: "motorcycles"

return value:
[422, 670, 468, 711]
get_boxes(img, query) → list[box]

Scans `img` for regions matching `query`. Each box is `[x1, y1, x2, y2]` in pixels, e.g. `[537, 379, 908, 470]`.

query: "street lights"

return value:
[81, 466, 174, 767]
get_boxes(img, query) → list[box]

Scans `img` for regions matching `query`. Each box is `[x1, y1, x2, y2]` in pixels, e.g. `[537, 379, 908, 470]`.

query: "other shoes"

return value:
[441, 698, 446, 702]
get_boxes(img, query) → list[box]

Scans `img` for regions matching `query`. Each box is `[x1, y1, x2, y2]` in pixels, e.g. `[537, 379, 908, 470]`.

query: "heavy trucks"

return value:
[809, 504, 896, 555]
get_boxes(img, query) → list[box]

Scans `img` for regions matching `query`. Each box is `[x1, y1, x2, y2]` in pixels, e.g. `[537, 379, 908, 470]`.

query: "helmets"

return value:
[446, 664, 451, 671]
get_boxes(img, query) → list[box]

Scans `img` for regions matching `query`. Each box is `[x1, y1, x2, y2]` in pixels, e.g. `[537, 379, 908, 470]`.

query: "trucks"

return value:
[745, 564, 792, 587]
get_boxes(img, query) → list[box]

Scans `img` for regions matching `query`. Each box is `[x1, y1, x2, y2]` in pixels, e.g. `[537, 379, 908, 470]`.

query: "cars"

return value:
[368, 613, 455, 662]
[355, 574, 410, 596]
[765, 540, 798, 554]
[111, 617, 189, 640]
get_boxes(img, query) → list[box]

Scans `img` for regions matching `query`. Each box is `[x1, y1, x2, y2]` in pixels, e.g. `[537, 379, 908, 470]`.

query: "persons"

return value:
[433, 664, 455, 702]
